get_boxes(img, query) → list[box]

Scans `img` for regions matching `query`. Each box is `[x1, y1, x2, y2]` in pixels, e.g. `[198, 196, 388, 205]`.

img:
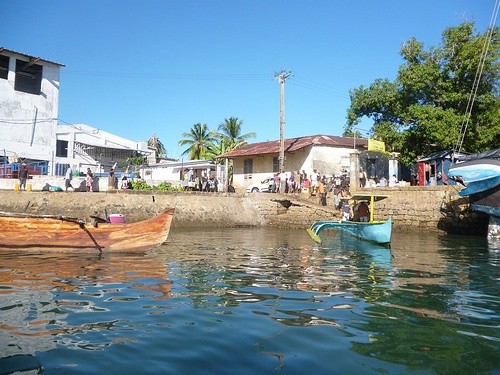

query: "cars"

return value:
[247, 177, 289, 192]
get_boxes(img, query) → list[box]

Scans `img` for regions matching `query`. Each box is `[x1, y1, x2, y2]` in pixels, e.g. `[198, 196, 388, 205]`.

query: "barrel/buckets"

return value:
[27, 184, 32, 191]
[14, 183, 19, 191]
[399, 181, 410, 187]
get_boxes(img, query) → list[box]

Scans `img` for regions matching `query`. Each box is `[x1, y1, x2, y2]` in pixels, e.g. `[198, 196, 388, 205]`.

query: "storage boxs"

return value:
[109, 213, 124, 223]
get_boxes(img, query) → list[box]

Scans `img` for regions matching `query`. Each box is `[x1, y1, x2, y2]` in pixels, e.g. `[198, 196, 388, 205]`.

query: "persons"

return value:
[64, 164, 75, 191]
[18, 163, 28, 190]
[181, 167, 216, 191]
[106, 169, 116, 188]
[271, 168, 370, 222]
[379, 175, 397, 187]
[86, 167, 94, 192]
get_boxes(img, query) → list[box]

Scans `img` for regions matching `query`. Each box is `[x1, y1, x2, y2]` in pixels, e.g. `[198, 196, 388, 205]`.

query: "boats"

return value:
[306, 195, 393, 245]
[0, 207, 176, 254]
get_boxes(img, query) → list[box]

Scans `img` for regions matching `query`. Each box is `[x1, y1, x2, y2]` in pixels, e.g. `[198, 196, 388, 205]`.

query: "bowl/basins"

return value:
[51, 186, 59, 191]
